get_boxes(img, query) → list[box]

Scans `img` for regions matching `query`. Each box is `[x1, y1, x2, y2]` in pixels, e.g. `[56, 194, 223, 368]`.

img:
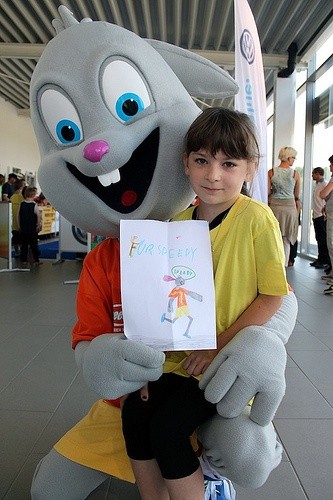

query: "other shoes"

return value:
[315, 264, 328, 269]
[331, 292, 333, 295]
[327, 280, 333, 284]
[322, 275, 333, 280]
[204, 478, 236, 500]
[324, 285, 333, 293]
[310, 260, 319, 266]
[288, 261, 293, 265]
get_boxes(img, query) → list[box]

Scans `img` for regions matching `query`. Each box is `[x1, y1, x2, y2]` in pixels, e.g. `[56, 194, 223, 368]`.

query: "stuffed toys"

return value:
[29, 7, 298, 500]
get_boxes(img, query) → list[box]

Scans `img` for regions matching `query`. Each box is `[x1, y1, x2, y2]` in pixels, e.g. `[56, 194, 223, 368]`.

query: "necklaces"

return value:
[196, 207, 228, 252]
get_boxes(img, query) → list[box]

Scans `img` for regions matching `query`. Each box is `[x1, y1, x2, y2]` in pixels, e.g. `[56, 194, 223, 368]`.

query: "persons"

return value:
[120, 105, 289, 500]
[320, 154, 333, 296]
[268, 146, 302, 267]
[17, 187, 43, 266]
[310, 167, 330, 269]
[0, 169, 50, 259]
